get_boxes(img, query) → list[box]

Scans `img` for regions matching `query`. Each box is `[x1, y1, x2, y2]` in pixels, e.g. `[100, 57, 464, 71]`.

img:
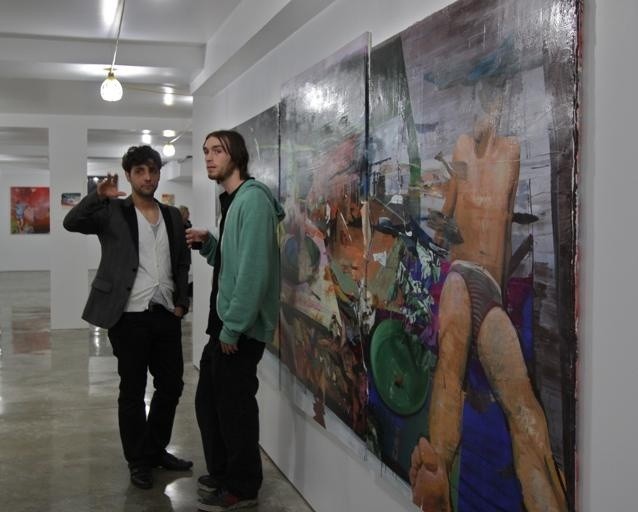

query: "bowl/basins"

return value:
[369, 317, 431, 417]
[281, 235, 322, 283]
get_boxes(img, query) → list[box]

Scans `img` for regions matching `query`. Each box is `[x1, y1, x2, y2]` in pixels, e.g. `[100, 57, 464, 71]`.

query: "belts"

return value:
[144, 305, 166, 313]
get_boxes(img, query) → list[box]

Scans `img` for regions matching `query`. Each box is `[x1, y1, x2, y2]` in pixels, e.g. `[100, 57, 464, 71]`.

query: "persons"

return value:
[61, 145, 196, 491]
[184, 128, 286, 512]
[401, 66, 570, 512]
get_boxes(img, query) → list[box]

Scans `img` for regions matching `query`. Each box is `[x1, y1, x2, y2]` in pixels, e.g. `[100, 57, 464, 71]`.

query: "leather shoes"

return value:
[130, 466, 154, 490]
[151, 450, 193, 471]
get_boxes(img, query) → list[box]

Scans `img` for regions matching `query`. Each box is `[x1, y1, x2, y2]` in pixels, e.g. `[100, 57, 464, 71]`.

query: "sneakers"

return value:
[195, 474, 259, 511]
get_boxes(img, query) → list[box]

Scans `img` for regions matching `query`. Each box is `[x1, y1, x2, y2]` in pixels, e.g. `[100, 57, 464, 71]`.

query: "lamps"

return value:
[100, 67, 123, 102]
